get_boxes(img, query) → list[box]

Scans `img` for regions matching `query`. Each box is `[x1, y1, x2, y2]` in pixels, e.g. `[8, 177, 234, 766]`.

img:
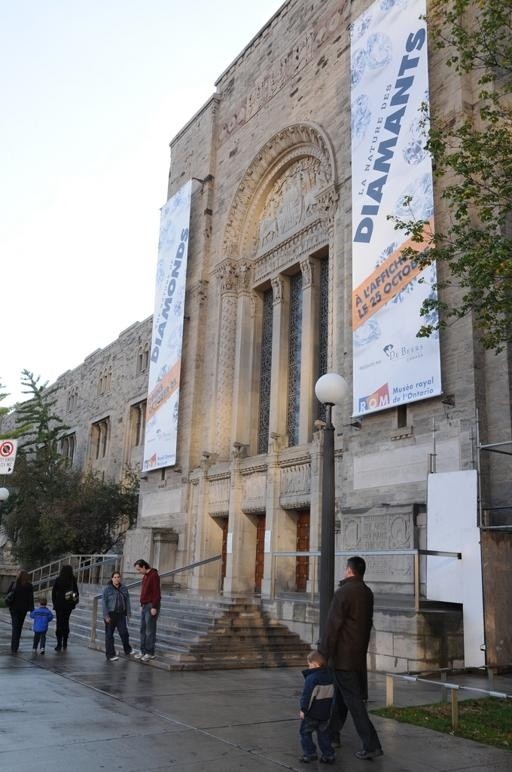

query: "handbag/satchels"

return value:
[63, 589, 80, 604]
[3, 580, 17, 607]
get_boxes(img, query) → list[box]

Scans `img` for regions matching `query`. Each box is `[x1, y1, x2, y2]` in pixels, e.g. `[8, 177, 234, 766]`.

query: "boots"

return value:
[54, 626, 70, 651]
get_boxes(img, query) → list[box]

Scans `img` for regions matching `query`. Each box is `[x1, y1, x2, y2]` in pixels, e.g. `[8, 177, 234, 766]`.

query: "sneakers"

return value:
[106, 647, 158, 662]
[298, 734, 384, 764]
[32, 647, 46, 655]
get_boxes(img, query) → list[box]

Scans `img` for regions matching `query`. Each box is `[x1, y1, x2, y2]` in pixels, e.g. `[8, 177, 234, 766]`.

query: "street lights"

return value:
[315, 374, 347, 656]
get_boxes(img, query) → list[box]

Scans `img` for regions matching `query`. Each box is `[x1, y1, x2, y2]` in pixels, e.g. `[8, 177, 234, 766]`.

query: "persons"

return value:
[294, 649, 340, 765]
[28, 596, 55, 656]
[3, 568, 34, 653]
[101, 572, 140, 662]
[313, 554, 386, 761]
[51, 563, 80, 653]
[131, 558, 162, 662]
